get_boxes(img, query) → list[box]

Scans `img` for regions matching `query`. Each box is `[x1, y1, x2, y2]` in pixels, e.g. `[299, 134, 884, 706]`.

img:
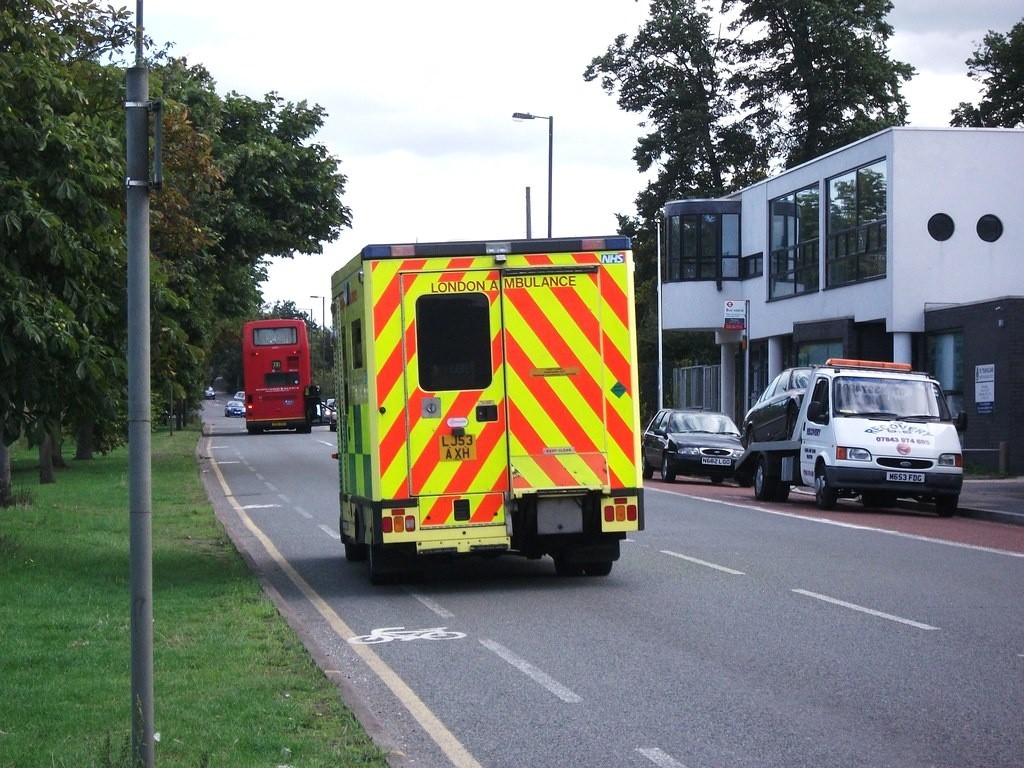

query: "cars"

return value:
[234, 390, 245, 406]
[224, 401, 245, 419]
[203, 388, 215, 400]
[738, 366, 825, 441]
[316, 398, 338, 431]
[642, 405, 747, 483]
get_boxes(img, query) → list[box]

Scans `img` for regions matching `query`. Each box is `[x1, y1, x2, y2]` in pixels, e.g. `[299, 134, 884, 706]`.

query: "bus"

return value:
[240, 319, 320, 433]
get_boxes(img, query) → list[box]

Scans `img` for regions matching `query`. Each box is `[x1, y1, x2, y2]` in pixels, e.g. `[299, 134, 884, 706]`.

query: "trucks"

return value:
[731, 356, 970, 519]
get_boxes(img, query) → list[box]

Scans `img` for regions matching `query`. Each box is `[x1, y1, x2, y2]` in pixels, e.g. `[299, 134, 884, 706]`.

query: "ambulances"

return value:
[330, 234, 645, 588]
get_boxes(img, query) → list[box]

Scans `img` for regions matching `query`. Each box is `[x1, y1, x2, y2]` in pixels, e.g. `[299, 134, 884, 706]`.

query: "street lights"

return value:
[311, 295, 326, 402]
[511, 112, 554, 238]
